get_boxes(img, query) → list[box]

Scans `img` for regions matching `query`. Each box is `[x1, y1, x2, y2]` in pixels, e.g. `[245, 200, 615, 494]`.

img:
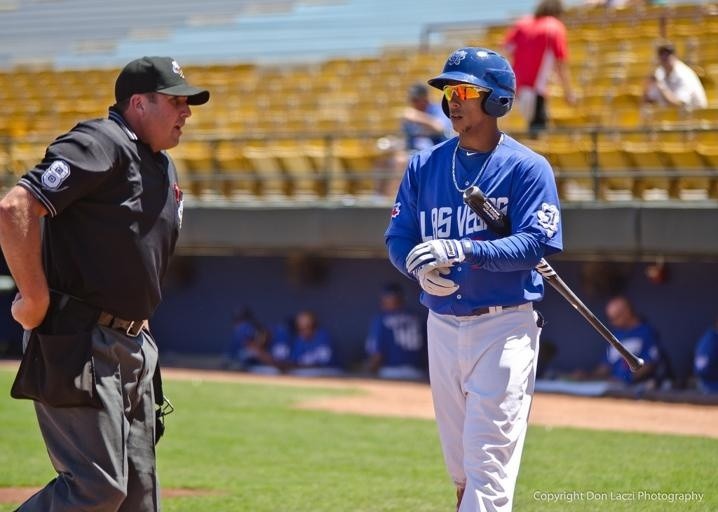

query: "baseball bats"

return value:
[463, 186, 646, 373]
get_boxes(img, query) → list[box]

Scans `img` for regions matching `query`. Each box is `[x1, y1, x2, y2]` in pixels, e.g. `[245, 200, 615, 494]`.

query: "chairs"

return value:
[1, 0, 718, 211]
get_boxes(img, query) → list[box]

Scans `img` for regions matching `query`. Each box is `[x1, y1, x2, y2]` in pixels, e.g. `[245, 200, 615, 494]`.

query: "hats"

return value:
[116, 55, 210, 105]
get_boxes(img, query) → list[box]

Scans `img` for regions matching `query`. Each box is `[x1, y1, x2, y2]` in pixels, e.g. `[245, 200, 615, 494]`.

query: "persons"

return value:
[400, 81, 453, 154]
[357, 282, 428, 380]
[693, 318, 717, 397]
[643, 43, 708, 111]
[1, 56, 211, 511]
[504, 0, 578, 138]
[383, 47, 564, 512]
[569, 297, 661, 386]
[221, 304, 343, 378]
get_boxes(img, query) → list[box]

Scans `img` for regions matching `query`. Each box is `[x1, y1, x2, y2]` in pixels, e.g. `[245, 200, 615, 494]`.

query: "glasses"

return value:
[444, 84, 490, 101]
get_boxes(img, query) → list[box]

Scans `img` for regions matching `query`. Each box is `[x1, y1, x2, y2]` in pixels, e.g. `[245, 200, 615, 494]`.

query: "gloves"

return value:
[404, 238, 472, 296]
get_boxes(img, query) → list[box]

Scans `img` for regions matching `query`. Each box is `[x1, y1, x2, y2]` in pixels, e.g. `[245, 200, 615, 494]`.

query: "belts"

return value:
[63, 300, 149, 336]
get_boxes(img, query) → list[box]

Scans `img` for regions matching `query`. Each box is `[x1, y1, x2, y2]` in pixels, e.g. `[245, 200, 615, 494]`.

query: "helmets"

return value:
[428, 44, 516, 117]
